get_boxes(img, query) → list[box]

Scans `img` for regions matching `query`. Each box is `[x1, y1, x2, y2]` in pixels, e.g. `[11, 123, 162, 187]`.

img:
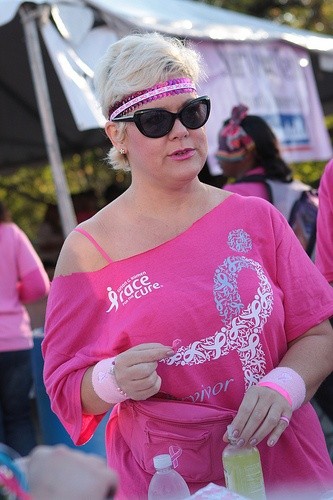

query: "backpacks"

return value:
[225, 175, 319, 264]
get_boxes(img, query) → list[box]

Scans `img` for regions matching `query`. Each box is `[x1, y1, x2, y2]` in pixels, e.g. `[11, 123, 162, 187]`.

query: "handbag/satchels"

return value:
[117, 396, 239, 483]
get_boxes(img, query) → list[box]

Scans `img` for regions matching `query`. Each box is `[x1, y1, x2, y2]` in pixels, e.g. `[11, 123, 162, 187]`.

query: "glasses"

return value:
[111, 95, 211, 138]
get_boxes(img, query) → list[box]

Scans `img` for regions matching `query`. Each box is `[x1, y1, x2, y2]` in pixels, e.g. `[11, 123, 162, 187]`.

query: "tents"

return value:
[0, 0, 333, 241]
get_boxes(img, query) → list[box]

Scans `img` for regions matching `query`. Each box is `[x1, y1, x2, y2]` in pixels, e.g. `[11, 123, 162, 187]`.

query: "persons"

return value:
[0, 444, 118, 500]
[217, 115, 318, 270]
[41, 34, 333, 499]
[0, 198, 50, 457]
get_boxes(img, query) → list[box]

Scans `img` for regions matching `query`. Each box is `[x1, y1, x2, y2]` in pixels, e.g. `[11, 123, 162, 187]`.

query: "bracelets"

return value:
[110, 356, 131, 399]
[256, 382, 292, 409]
[257, 367, 306, 412]
[92, 356, 129, 404]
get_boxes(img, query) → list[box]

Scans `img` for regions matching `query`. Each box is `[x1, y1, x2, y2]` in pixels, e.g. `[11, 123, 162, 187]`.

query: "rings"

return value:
[280, 416, 289, 426]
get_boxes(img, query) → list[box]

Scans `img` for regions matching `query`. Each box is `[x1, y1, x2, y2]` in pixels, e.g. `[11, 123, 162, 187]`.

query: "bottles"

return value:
[147, 453, 190, 500]
[222, 424, 266, 500]
